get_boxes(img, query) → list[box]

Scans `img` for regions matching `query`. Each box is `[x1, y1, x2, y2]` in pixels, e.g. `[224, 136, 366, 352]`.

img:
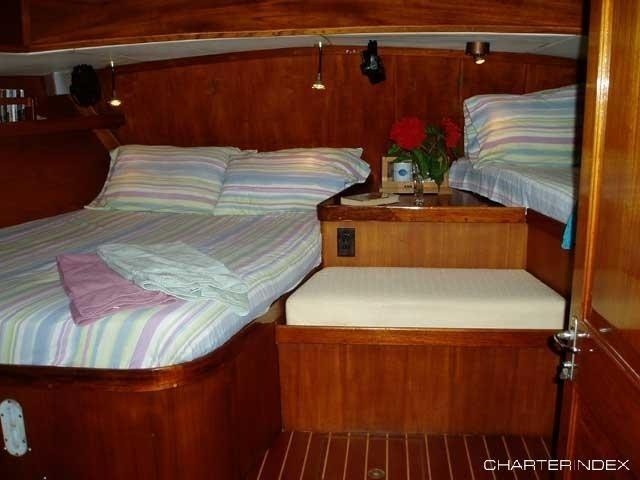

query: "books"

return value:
[339, 192, 401, 207]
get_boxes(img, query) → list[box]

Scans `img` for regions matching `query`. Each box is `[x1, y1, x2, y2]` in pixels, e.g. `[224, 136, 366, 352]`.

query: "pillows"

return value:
[462, 85, 577, 168]
[83, 145, 371, 216]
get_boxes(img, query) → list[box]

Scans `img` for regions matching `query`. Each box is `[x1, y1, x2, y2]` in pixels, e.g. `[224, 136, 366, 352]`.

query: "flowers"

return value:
[389, 118, 460, 185]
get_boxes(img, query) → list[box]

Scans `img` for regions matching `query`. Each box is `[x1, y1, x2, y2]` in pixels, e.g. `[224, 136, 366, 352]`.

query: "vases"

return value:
[411, 160, 424, 205]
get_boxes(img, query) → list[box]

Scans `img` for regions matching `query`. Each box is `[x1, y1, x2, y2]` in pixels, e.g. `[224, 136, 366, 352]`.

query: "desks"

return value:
[316, 175, 528, 270]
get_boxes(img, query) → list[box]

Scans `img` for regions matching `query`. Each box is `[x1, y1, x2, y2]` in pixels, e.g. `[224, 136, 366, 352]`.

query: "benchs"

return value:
[275, 266, 567, 439]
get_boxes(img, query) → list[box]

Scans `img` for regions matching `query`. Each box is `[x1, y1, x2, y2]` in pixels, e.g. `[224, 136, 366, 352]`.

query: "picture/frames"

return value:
[379, 156, 451, 194]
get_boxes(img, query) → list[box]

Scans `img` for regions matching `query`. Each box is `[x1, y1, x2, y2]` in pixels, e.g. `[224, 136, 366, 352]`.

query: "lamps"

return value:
[311, 40, 326, 90]
[466, 41, 489, 64]
[108, 60, 122, 107]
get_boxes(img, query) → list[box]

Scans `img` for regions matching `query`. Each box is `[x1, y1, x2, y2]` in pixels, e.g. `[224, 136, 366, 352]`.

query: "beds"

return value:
[449, 158, 577, 299]
[0, 208, 323, 480]
